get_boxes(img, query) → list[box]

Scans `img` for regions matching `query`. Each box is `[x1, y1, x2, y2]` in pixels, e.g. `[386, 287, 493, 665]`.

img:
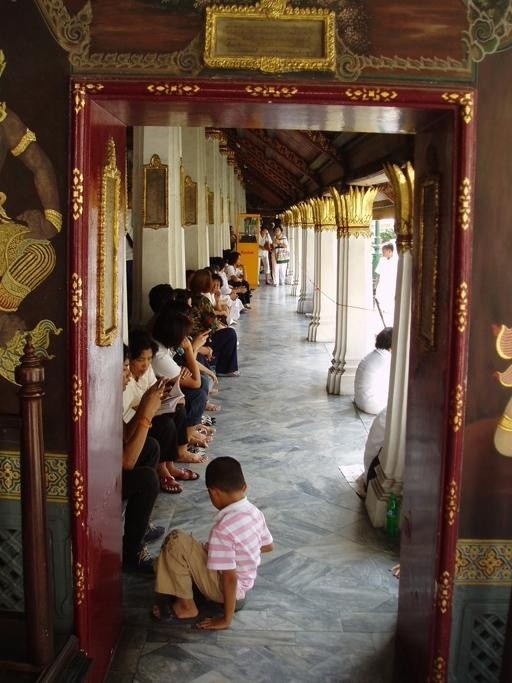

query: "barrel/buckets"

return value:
[151, 601, 203, 625]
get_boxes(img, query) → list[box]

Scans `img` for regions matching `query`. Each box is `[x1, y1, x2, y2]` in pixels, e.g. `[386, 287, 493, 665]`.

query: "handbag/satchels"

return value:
[44, 209, 63, 232]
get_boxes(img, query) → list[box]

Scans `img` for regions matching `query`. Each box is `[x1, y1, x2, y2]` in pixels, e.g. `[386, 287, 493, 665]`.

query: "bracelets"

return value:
[139, 420, 152, 427]
[44, 209, 63, 232]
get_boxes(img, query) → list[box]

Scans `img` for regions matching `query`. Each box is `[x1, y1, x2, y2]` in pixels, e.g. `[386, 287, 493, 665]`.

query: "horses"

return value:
[157, 379, 164, 389]
[187, 335, 194, 343]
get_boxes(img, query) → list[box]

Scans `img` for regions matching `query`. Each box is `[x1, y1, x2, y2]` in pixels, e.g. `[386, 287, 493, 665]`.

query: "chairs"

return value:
[216, 370, 240, 377]
[144, 520, 165, 544]
[136, 543, 157, 578]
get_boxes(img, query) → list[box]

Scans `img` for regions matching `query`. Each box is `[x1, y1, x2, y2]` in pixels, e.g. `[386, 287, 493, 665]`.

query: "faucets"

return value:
[186, 446, 205, 456]
[200, 415, 216, 426]
[159, 475, 183, 493]
[171, 468, 199, 481]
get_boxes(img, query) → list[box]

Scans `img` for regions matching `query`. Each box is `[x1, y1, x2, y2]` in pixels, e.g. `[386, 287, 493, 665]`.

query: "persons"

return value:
[123, 343, 172, 570]
[354, 327, 392, 415]
[259, 226, 288, 286]
[152, 456, 274, 629]
[149, 249, 252, 461]
[375, 244, 398, 328]
[0, 50, 62, 346]
[364, 406, 386, 487]
[129, 334, 200, 493]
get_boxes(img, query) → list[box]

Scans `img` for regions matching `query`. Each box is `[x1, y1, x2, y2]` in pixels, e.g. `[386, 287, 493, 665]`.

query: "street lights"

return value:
[275, 247, 290, 264]
